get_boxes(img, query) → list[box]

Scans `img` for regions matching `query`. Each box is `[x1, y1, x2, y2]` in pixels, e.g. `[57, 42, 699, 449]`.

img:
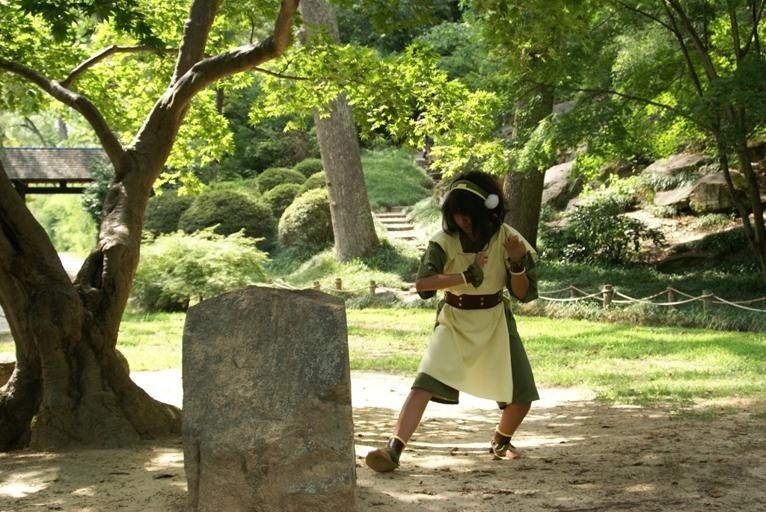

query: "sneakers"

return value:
[368, 449, 400, 471]
[491, 437, 520, 460]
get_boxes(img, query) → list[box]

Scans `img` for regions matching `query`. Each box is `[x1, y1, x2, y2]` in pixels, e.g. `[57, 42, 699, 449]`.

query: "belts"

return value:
[444, 289, 504, 310]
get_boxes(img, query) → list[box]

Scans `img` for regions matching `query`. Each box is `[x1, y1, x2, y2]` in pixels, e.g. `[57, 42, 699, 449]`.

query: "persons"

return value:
[420, 133, 433, 161]
[363, 168, 541, 476]
